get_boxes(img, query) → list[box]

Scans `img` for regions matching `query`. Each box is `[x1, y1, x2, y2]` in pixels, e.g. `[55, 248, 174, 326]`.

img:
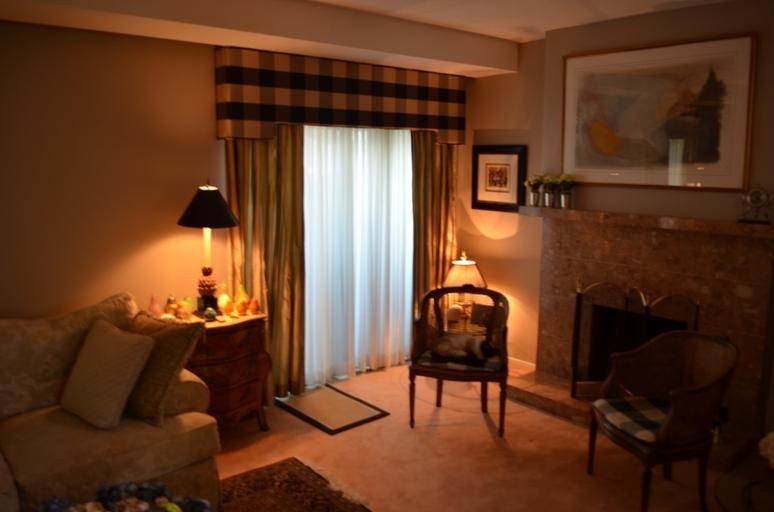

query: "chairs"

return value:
[587, 331, 741, 512]
[409, 285, 508, 437]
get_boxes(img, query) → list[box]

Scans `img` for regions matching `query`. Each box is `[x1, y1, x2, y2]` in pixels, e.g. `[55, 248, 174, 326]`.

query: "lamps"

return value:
[443, 249, 487, 332]
[177, 180, 240, 315]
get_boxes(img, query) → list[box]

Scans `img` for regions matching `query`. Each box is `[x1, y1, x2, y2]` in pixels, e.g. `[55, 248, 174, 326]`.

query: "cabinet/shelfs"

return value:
[159, 310, 271, 430]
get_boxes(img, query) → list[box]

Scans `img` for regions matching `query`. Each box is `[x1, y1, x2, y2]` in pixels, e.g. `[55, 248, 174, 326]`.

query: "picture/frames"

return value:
[561, 31, 754, 193]
[471, 144, 526, 211]
[737, 186, 773, 223]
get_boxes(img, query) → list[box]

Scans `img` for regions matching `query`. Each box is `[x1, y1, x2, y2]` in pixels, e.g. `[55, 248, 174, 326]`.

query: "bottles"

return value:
[217, 283, 259, 316]
[204, 307, 217, 321]
[165, 295, 178, 316]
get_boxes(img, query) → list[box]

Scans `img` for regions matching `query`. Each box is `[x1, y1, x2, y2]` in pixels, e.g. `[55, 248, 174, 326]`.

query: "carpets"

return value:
[276, 380, 390, 435]
[220, 458, 371, 512]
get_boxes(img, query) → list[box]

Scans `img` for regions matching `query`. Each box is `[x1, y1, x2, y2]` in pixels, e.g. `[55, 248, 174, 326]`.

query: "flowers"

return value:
[543, 175, 559, 192]
[524, 175, 542, 192]
[558, 174, 576, 194]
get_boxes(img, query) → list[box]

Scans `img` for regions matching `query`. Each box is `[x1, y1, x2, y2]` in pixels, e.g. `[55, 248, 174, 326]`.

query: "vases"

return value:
[560, 193, 572, 209]
[529, 192, 539, 207]
[544, 192, 554, 208]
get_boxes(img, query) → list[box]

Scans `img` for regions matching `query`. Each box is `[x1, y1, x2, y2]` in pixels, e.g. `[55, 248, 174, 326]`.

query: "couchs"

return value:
[1, 292, 222, 512]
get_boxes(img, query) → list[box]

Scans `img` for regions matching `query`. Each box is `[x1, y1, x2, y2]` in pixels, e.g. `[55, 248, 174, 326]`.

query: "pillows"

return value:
[129, 312, 205, 428]
[62, 317, 154, 431]
